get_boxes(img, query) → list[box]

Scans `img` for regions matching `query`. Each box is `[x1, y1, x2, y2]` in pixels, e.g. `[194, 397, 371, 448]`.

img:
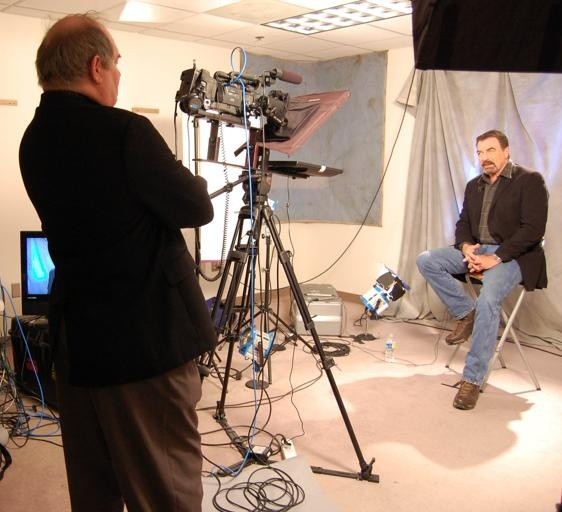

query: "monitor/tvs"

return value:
[19, 231, 55, 316]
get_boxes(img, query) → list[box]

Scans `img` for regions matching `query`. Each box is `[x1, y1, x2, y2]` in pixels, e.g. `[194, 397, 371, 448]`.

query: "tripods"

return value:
[197, 172, 380, 482]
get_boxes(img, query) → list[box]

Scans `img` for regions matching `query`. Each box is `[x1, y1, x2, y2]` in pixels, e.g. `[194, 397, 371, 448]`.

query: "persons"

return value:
[417, 130, 548, 410]
[18, 9, 214, 512]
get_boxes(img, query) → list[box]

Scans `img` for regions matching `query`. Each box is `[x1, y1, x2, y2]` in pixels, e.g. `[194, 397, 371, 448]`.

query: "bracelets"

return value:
[493, 253, 502, 262]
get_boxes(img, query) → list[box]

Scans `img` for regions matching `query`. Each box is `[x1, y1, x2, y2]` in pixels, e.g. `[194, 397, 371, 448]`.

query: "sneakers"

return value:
[446, 309, 475, 344]
[453, 380, 480, 410]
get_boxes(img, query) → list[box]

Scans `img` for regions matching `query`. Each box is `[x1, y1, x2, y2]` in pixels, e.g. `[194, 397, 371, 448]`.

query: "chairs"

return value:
[444, 235, 546, 393]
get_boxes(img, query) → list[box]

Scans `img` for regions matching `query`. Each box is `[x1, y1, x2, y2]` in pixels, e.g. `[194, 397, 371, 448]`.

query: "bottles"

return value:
[385, 333, 395, 364]
